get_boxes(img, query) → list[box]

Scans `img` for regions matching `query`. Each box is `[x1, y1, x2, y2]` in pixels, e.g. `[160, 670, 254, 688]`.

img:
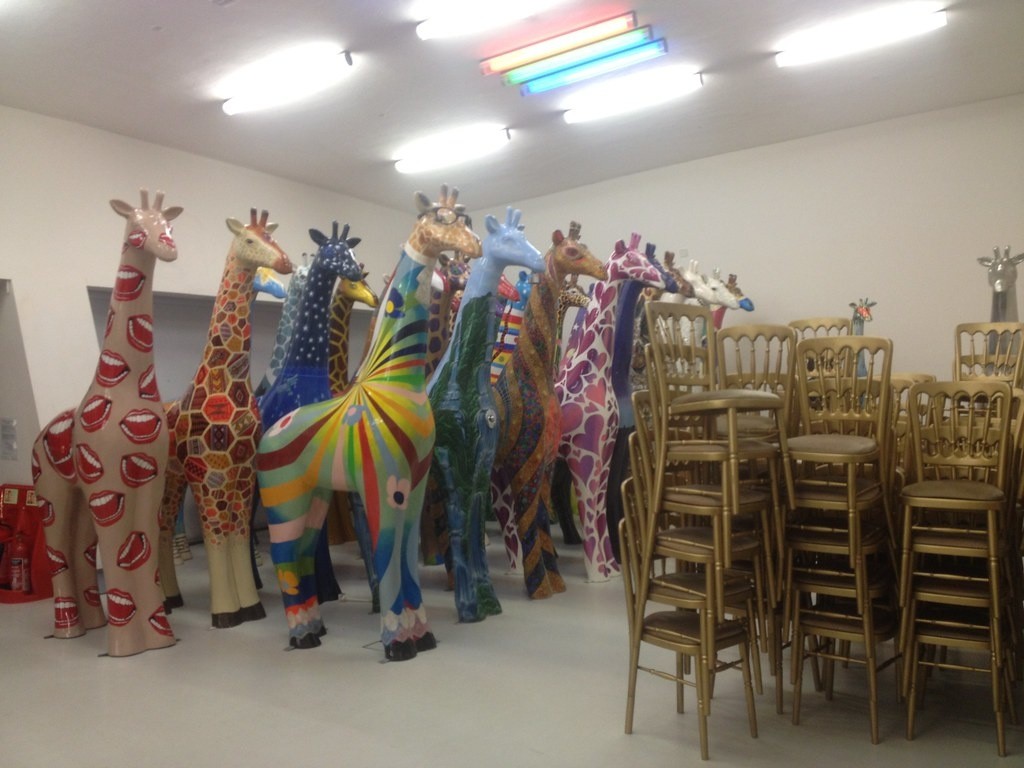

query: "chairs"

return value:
[606, 294, 1024, 758]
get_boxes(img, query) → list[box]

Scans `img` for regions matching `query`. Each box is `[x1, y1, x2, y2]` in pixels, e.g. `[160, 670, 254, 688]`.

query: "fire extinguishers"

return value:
[10, 528, 32, 596]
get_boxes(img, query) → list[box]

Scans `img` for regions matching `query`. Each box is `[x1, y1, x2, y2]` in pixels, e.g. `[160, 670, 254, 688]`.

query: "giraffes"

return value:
[33, 182, 754, 664]
